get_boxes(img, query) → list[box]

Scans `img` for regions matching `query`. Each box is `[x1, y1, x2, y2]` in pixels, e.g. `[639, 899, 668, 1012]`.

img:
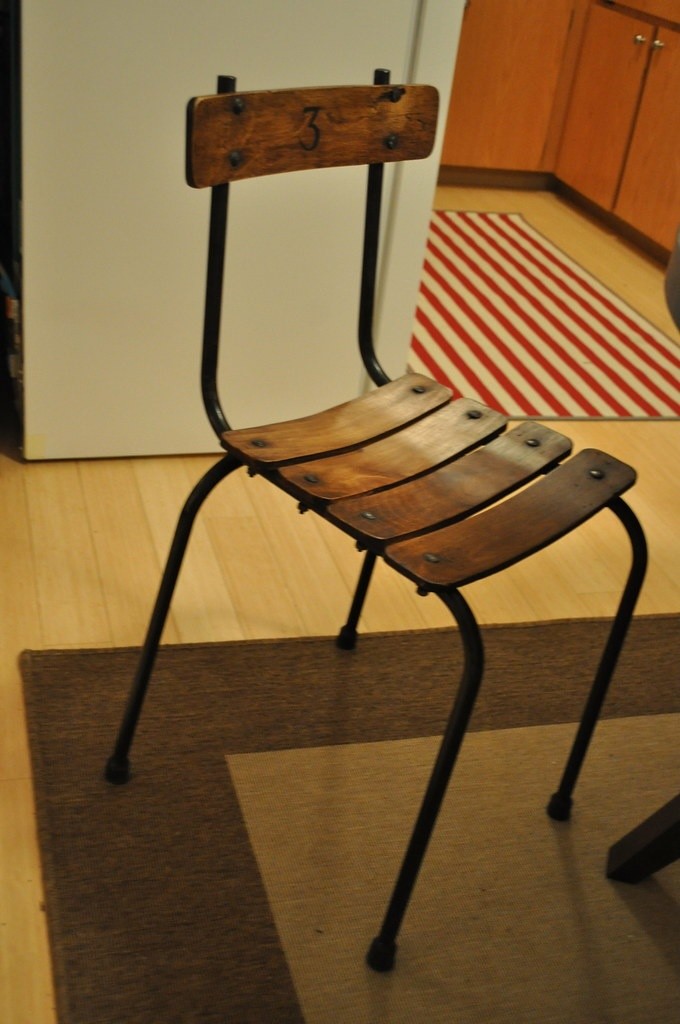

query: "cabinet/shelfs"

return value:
[556, 0, 680, 259]
[440, 0, 579, 172]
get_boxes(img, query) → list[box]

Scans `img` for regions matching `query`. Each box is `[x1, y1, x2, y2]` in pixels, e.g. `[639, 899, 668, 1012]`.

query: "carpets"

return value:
[404, 211, 679, 421]
[18, 613, 680, 1024]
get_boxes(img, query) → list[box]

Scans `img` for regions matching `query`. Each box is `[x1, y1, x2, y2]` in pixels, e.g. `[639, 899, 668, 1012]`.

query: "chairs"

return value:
[108, 68, 648, 977]
